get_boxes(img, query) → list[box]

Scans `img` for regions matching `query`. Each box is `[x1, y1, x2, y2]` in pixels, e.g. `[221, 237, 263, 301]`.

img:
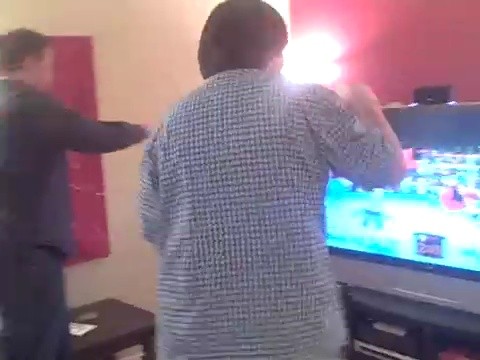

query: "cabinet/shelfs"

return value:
[337, 282, 480, 359]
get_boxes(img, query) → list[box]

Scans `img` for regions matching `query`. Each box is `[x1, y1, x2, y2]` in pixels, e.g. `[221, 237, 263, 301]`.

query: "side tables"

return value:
[69, 298, 156, 360]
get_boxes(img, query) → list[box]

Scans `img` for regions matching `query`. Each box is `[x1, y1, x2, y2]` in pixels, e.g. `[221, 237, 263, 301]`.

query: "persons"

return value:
[1, 28, 158, 360]
[138, 0, 407, 360]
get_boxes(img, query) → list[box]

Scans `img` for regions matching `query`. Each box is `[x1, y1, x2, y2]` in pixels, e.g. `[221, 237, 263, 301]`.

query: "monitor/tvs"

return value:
[315, 100, 480, 317]
